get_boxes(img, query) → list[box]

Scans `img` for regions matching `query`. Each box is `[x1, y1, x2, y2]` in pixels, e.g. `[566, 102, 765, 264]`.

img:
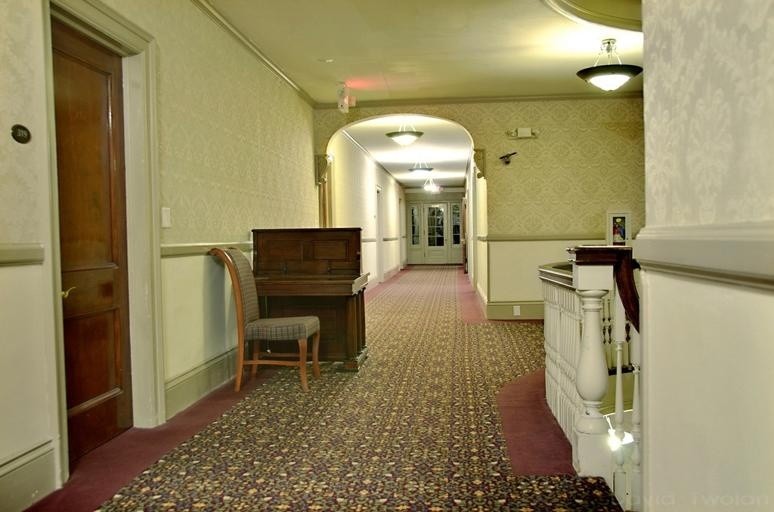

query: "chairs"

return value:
[206, 245, 324, 393]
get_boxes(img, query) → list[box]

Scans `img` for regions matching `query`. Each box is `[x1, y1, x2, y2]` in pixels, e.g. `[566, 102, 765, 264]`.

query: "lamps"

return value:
[574, 38, 644, 94]
[384, 123, 424, 146]
